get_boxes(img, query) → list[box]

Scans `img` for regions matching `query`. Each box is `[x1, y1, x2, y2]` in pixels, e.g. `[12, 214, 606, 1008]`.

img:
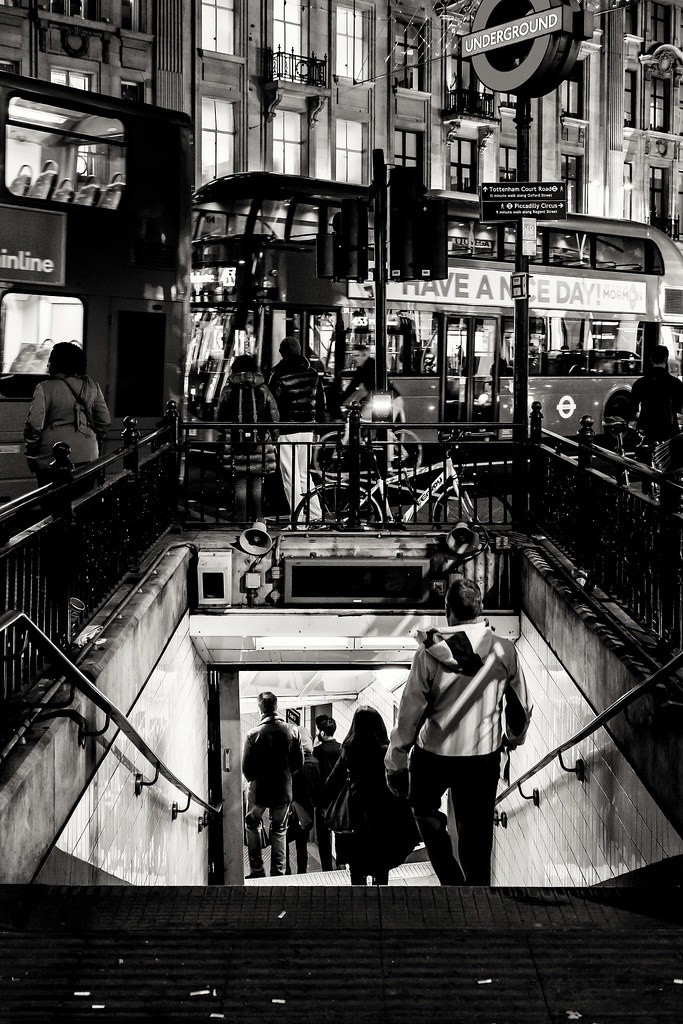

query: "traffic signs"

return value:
[479, 181, 568, 224]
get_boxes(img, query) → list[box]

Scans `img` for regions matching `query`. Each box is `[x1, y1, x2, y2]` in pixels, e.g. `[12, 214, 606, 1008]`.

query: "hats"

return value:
[231, 355, 257, 373]
[279, 338, 300, 357]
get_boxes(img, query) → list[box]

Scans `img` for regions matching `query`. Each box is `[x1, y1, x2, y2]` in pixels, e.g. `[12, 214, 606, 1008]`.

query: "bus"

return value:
[0, 69, 199, 536]
[176, 167, 683, 518]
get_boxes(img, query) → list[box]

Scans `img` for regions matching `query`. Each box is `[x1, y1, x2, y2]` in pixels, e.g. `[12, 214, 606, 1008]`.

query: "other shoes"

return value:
[282, 525, 306, 530]
[245, 871, 265, 879]
[308, 519, 325, 529]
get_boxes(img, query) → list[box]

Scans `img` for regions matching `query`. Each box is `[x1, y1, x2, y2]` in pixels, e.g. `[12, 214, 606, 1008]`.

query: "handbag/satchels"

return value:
[291, 802, 313, 831]
[325, 756, 355, 835]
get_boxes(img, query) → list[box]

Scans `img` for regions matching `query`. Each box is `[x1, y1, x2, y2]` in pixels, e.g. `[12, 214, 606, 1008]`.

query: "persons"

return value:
[386, 579, 533, 886]
[282, 724, 319, 875]
[213, 354, 281, 522]
[25, 341, 112, 486]
[336, 343, 404, 455]
[242, 692, 305, 878]
[307, 714, 347, 872]
[267, 337, 327, 531]
[626, 345, 683, 517]
[423, 353, 436, 374]
[315, 707, 422, 885]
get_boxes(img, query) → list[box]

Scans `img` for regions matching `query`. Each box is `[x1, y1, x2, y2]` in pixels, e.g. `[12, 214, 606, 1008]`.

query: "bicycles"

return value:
[290, 442, 510, 532]
[311, 398, 424, 487]
[601, 415, 663, 504]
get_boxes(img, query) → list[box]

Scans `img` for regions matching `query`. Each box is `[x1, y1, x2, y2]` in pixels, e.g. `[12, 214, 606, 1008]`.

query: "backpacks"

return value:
[227, 381, 267, 450]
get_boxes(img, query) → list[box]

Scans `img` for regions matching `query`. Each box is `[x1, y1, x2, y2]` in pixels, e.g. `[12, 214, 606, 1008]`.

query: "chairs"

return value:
[551, 351, 641, 375]
[10, 160, 125, 211]
